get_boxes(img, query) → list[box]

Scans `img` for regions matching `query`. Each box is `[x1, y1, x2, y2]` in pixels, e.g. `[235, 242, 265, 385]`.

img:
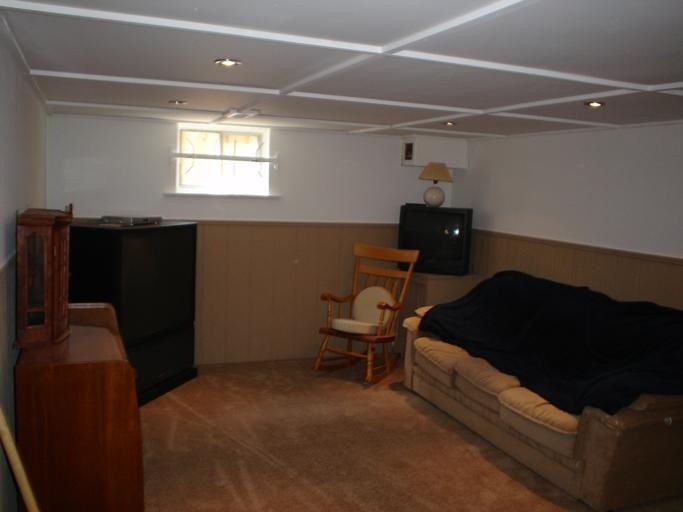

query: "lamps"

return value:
[419, 162, 453, 206]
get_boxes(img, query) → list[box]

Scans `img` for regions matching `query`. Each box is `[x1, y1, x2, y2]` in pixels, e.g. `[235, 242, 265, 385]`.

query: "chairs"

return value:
[314, 243, 419, 385]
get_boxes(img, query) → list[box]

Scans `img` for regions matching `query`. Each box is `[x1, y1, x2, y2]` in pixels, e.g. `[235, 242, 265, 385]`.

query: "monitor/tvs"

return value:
[68, 218, 197, 350]
[398, 203, 473, 275]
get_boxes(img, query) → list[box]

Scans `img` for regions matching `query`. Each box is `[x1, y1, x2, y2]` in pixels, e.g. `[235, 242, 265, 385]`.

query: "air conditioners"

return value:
[401, 136, 468, 169]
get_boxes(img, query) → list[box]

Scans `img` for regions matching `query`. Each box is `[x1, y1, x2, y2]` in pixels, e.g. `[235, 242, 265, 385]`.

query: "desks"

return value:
[68, 220, 197, 407]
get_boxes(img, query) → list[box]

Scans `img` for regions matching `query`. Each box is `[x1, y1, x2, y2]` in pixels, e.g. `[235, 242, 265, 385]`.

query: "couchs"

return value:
[404, 268, 683, 512]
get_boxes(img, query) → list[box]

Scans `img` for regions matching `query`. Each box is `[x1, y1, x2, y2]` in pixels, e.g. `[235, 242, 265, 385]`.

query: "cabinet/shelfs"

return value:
[400, 263, 483, 359]
[15, 207, 142, 512]
